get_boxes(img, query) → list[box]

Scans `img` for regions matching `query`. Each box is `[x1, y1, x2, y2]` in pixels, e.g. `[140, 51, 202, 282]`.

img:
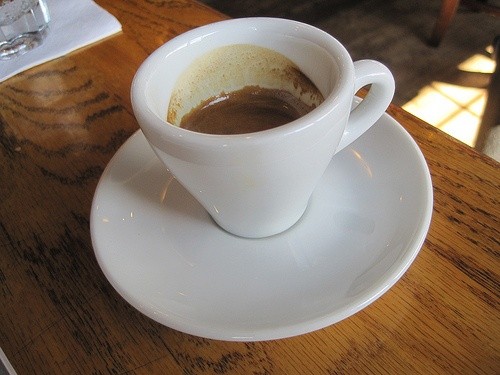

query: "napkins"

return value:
[0, 0, 122, 83]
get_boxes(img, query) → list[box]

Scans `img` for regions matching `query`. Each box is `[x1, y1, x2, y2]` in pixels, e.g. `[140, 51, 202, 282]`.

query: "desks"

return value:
[0, 0, 500, 375]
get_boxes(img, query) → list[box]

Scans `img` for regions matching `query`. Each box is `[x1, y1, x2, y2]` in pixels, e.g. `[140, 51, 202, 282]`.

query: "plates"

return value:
[89, 95, 434, 342]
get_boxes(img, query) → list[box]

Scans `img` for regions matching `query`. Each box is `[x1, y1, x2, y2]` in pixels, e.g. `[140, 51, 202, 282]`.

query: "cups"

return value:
[129, 17, 395, 239]
[0, 0, 51, 61]
